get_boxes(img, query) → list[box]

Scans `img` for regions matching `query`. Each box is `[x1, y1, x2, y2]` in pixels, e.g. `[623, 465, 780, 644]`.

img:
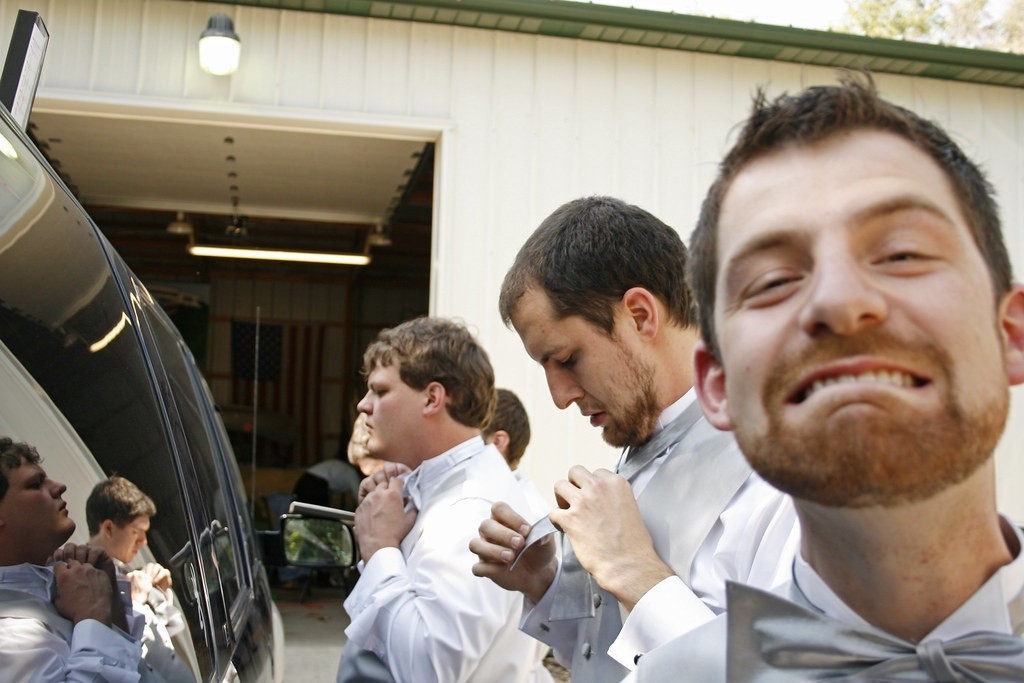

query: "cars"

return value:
[0, 104, 364, 683]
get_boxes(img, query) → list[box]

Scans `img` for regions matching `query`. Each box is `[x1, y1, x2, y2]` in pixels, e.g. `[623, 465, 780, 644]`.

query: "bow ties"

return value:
[727, 548, 1024, 683]
[505, 397, 701, 622]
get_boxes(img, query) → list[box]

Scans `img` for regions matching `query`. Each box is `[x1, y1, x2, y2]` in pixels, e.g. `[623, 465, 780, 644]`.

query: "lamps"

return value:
[197, 14, 241, 78]
[367, 224, 393, 246]
[185, 234, 372, 265]
[166, 211, 194, 234]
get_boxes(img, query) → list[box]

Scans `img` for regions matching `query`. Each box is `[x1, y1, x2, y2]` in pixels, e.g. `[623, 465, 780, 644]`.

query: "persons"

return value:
[1, 438, 197, 683]
[464, 200, 800, 683]
[624, 82, 1024, 682]
[340, 317, 559, 683]
